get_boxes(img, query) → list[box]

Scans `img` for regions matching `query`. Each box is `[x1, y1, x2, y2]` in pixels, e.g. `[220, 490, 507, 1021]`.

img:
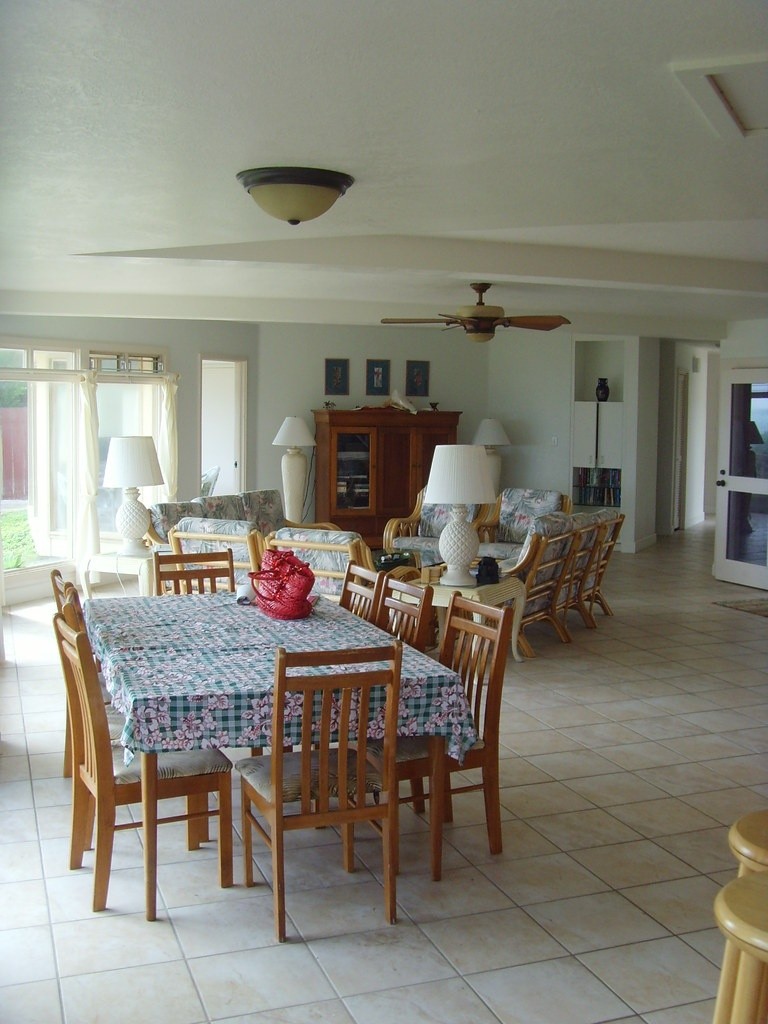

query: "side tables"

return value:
[389, 577, 527, 662]
[79, 551, 176, 601]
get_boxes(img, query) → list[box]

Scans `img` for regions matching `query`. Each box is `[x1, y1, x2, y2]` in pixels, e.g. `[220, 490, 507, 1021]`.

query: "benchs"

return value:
[469, 508, 626, 660]
[147, 490, 343, 596]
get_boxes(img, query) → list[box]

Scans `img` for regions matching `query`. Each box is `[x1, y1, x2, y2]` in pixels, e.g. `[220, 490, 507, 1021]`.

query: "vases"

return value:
[595, 377, 609, 401]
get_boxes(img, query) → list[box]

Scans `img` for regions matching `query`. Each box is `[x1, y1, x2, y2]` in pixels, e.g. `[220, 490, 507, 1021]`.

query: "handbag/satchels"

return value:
[248, 549, 315, 620]
[476, 556, 499, 584]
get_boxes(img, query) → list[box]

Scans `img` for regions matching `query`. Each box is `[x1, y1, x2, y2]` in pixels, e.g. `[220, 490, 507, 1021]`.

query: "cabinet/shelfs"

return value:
[573, 486, 621, 508]
[311, 410, 466, 550]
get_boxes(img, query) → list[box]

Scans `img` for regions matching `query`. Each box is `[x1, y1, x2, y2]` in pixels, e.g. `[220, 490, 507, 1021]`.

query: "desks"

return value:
[83, 591, 479, 925]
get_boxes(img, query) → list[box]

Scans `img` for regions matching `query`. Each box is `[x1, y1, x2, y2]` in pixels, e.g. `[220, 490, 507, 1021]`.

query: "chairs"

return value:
[168, 517, 437, 648]
[52, 548, 513, 944]
[383, 484, 573, 570]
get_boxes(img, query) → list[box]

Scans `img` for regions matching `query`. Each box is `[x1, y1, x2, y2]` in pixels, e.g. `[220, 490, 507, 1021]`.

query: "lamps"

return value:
[742, 421, 764, 533]
[471, 417, 512, 496]
[236, 167, 353, 226]
[103, 436, 164, 557]
[423, 444, 497, 587]
[273, 416, 317, 523]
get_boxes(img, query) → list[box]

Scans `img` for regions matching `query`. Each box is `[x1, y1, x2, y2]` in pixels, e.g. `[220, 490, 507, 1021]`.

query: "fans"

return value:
[381, 283, 571, 343]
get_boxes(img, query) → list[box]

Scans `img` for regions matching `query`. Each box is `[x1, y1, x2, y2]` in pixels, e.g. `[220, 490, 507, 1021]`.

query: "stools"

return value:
[712, 810, 768, 1024]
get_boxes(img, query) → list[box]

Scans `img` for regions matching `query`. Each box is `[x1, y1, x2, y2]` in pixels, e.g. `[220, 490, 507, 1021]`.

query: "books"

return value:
[578, 468, 621, 507]
[237, 584, 258, 606]
[306, 595, 320, 607]
[337, 451, 369, 493]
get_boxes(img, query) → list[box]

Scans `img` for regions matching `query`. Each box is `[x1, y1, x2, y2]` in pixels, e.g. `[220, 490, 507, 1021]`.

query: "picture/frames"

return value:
[325, 359, 349, 395]
[366, 359, 391, 395]
[405, 360, 430, 397]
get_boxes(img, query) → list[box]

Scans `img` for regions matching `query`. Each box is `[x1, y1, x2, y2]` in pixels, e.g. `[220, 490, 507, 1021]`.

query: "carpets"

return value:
[711, 598, 768, 619]
